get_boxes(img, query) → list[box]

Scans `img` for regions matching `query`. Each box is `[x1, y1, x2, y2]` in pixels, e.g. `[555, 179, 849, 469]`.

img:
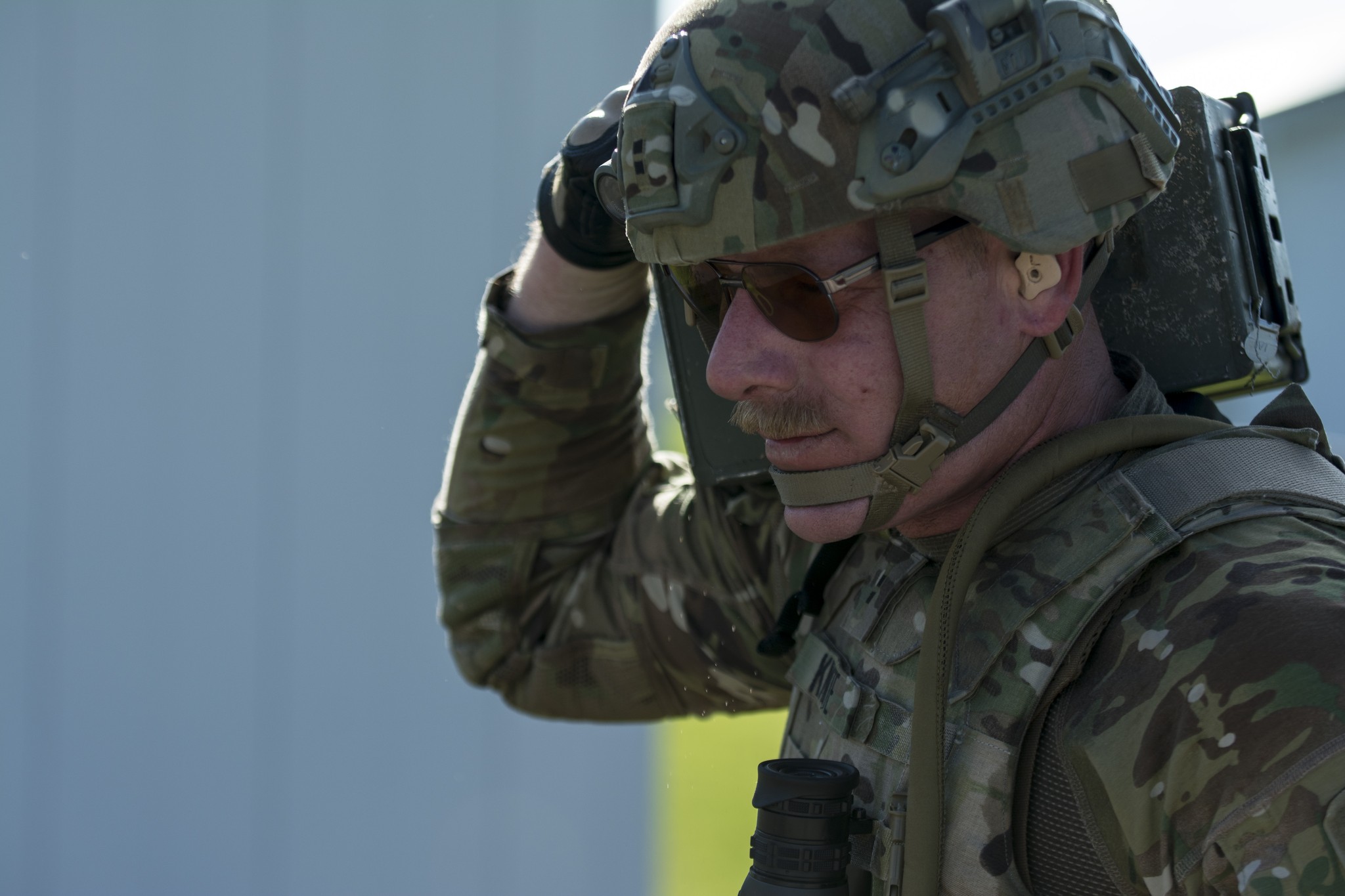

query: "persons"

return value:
[426, 1, 1345, 896]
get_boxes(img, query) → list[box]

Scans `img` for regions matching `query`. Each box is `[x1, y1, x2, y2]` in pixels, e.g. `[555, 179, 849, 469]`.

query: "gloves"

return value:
[537, 81, 637, 274]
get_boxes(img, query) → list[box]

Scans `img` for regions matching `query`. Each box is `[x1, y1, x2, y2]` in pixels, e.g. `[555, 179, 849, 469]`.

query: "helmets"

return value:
[592, 1, 1184, 265]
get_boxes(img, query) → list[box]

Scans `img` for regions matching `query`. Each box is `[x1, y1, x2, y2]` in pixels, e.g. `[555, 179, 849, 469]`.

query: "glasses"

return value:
[667, 216, 972, 343]
